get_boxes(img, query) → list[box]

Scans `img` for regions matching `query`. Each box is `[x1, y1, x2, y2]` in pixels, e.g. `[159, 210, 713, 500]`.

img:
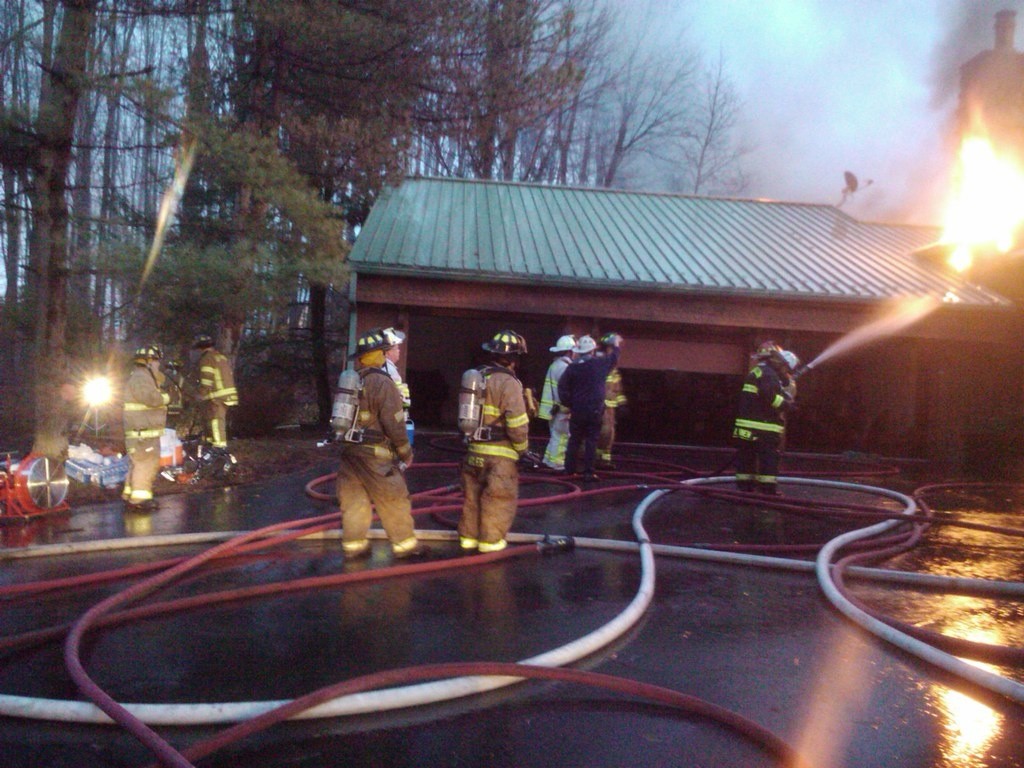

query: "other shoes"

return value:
[565, 466, 586, 473]
[600, 462, 616, 470]
[344, 543, 373, 563]
[121, 497, 161, 515]
[761, 483, 777, 494]
[592, 459, 602, 468]
[736, 479, 755, 492]
[393, 544, 432, 561]
[584, 473, 601, 483]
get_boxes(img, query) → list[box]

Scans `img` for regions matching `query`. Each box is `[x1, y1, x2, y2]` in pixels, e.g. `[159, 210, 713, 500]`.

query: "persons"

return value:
[326, 327, 433, 565]
[751, 341, 797, 484]
[557, 333, 620, 483]
[191, 334, 239, 451]
[537, 334, 576, 475]
[456, 328, 530, 553]
[592, 331, 629, 471]
[730, 348, 802, 496]
[120, 344, 179, 515]
[378, 327, 412, 473]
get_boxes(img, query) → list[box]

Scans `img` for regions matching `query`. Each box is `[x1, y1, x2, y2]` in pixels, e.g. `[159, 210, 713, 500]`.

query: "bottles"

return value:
[405, 419, 414, 445]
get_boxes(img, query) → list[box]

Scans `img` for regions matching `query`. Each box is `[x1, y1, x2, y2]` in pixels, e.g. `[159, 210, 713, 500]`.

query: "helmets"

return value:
[756, 341, 778, 356]
[383, 327, 406, 346]
[132, 343, 163, 361]
[480, 329, 528, 356]
[572, 336, 597, 354]
[549, 335, 576, 352]
[349, 326, 392, 359]
[599, 332, 623, 345]
[190, 334, 213, 348]
[779, 350, 799, 370]
